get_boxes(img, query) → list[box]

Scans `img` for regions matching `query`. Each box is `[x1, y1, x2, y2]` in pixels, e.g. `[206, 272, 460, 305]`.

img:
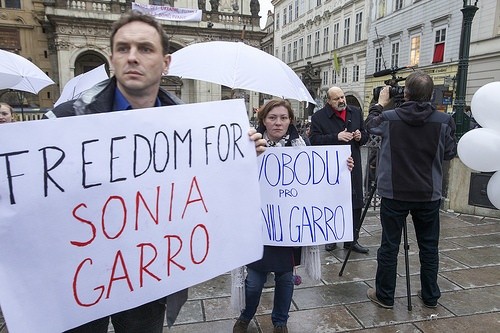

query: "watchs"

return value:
[375, 103, 383, 108]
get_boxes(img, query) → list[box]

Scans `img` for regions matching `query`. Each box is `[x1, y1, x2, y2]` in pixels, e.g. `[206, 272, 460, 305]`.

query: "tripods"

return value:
[339, 176, 412, 311]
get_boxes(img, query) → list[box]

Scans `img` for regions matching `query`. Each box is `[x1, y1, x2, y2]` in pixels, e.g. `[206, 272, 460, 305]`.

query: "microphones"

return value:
[373, 65, 414, 77]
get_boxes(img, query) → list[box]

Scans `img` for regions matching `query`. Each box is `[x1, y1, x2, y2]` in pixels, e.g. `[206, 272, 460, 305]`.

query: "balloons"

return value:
[486, 172, 500, 209]
[457, 128, 500, 172]
[471, 81, 500, 130]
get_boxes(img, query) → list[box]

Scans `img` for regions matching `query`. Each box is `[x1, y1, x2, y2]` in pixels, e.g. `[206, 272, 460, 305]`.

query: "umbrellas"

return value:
[161, 41, 317, 106]
[0, 50, 56, 96]
[52, 64, 110, 108]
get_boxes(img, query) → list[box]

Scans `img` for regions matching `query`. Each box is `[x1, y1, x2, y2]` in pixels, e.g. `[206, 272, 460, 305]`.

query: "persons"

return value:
[310, 86, 369, 254]
[233, 98, 354, 333]
[365, 73, 458, 308]
[0, 102, 14, 124]
[41, 9, 266, 333]
[295, 119, 313, 147]
[302, 61, 316, 79]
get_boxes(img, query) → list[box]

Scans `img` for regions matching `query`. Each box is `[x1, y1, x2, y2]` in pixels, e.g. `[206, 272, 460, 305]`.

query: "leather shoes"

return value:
[325, 243, 336, 251]
[343, 243, 370, 253]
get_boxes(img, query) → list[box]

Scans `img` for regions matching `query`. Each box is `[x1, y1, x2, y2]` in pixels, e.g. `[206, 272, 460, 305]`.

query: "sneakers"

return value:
[273, 325, 288, 333]
[233, 317, 249, 333]
[417, 289, 438, 309]
[366, 288, 395, 309]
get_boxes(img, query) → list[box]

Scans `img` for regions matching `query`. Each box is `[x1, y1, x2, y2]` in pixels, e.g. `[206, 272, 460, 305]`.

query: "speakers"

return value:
[468, 172, 499, 210]
